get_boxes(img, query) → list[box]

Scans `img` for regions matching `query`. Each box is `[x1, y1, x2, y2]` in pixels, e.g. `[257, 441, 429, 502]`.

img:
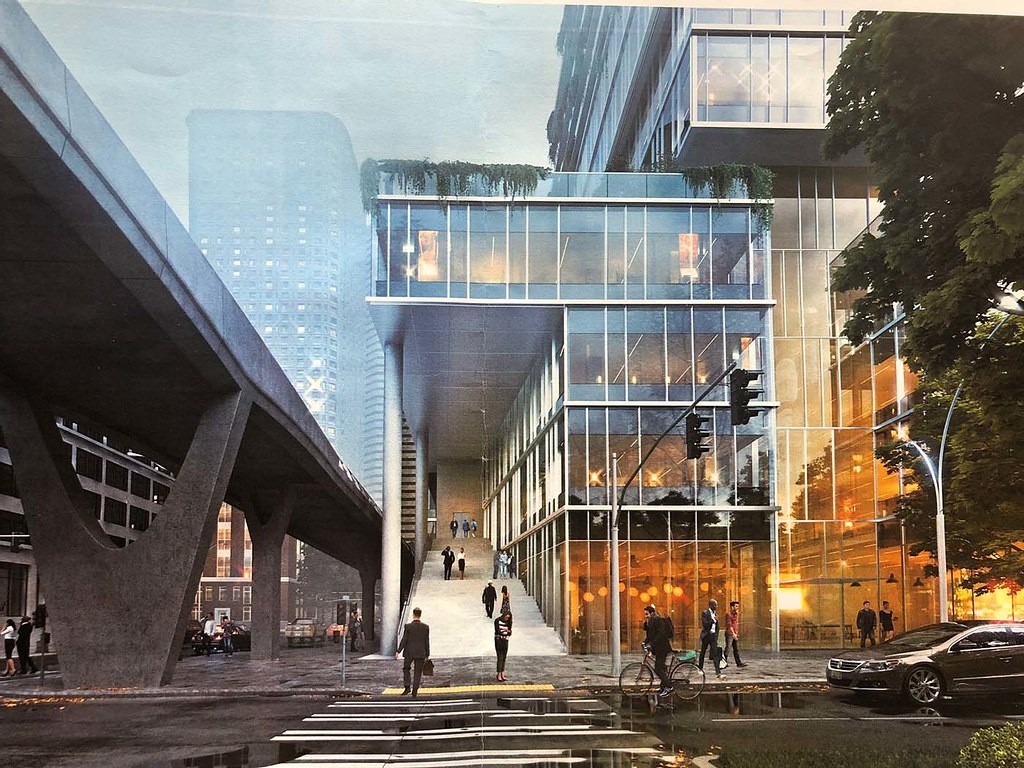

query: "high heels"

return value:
[11, 669, 17, 676]
[497, 675, 503, 682]
[1, 670, 10, 676]
[501, 675, 507, 681]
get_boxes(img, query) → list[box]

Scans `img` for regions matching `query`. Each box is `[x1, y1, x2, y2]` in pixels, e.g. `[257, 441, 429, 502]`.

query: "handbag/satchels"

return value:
[467, 526, 470, 531]
[709, 647, 723, 660]
[484, 587, 488, 602]
[472, 526, 476, 532]
[423, 660, 434, 675]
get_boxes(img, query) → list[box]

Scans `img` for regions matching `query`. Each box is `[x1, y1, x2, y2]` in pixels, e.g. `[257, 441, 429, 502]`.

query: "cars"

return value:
[326, 622, 350, 637]
[826, 619, 1024, 707]
[182, 619, 251, 654]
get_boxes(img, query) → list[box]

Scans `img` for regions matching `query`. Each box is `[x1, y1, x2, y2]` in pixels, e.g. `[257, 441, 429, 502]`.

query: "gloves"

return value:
[641, 641, 646, 648]
[647, 645, 652, 651]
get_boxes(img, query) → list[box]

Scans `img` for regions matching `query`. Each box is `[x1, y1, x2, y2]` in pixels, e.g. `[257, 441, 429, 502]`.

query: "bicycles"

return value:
[619, 641, 706, 700]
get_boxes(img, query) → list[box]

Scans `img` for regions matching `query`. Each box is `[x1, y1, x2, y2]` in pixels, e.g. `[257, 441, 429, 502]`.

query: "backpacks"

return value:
[660, 617, 674, 638]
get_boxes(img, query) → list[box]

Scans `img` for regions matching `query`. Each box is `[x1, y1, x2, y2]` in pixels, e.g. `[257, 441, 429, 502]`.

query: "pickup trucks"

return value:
[284, 617, 327, 645]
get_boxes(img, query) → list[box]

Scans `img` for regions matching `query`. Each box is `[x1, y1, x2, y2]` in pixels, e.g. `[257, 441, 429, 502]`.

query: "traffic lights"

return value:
[686, 413, 715, 459]
[337, 601, 347, 626]
[730, 369, 766, 425]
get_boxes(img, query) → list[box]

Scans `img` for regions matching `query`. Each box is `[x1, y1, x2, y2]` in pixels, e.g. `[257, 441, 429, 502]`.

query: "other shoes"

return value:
[225, 653, 229, 655]
[18, 670, 27, 674]
[412, 690, 417, 697]
[401, 688, 410, 696]
[350, 648, 358, 652]
[206, 653, 210, 657]
[716, 674, 726, 678]
[28, 670, 36, 674]
[737, 663, 748, 668]
[489, 613, 492, 618]
[228, 655, 232, 658]
[659, 687, 675, 697]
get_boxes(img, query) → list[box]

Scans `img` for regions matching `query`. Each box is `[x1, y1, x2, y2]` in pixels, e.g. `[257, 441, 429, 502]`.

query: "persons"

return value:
[348, 610, 360, 652]
[0, 619, 18, 676]
[723, 601, 748, 667]
[199, 612, 216, 657]
[394, 607, 431, 697]
[879, 600, 898, 642]
[440, 516, 516, 581]
[220, 616, 236, 657]
[482, 580, 497, 619]
[697, 599, 727, 679]
[857, 601, 878, 648]
[494, 611, 513, 682]
[641, 606, 675, 697]
[16, 616, 38, 675]
[499, 586, 511, 615]
[356, 617, 366, 650]
